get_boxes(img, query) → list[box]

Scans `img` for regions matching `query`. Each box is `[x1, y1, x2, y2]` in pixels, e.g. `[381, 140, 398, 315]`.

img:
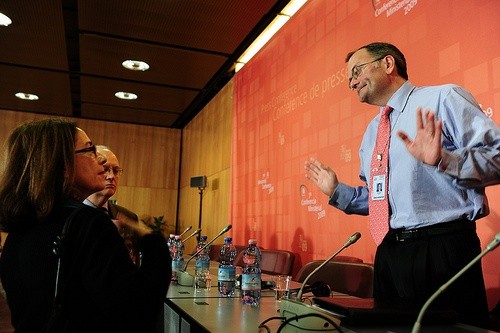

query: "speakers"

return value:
[190, 175, 206, 188]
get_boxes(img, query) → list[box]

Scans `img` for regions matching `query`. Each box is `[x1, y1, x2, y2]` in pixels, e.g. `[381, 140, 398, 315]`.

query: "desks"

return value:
[164, 254, 498, 333]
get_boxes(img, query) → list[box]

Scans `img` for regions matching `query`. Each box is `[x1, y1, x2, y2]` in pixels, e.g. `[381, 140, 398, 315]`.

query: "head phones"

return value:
[282, 280, 331, 298]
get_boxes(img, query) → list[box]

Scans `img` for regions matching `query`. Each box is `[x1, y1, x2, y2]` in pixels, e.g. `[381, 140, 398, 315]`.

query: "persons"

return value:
[377, 183, 382, 191]
[304, 42, 500, 333]
[0, 118, 172, 333]
[83, 145, 134, 250]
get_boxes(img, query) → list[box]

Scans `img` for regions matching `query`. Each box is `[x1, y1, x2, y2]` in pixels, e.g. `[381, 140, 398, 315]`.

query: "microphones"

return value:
[176, 224, 231, 287]
[281, 231, 362, 330]
[180, 226, 192, 236]
[411, 231, 500, 333]
[182, 228, 200, 244]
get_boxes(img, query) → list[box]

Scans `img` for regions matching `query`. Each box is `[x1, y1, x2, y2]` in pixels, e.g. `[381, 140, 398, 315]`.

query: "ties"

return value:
[368, 105, 393, 247]
[95, 207, 107, 213]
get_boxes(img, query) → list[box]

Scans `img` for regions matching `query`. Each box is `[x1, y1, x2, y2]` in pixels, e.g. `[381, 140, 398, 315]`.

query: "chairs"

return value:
[233, 248, 293, 276]
[295, 260, 375, 298]
[192, 244, 220, 261]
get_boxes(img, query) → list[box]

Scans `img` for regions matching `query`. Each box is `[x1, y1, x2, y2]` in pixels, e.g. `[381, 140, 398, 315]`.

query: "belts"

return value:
[384, 219, 476, 242]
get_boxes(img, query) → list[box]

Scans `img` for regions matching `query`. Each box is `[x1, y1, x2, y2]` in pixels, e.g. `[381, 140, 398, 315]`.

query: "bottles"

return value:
[218, 238, 236, 297]
[195, 236, 210, 275]
[167, 234, 185, 271]
[242, 239, 262, 305]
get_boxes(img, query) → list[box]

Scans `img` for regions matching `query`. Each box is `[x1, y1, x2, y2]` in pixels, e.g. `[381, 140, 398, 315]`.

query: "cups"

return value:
[273, 275, 292, 290]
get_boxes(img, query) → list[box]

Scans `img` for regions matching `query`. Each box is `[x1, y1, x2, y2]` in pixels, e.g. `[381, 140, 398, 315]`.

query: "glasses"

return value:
[74, 144, 96, 157]
[103, 166, 123, 176]
[349, 54, 396, 91]
